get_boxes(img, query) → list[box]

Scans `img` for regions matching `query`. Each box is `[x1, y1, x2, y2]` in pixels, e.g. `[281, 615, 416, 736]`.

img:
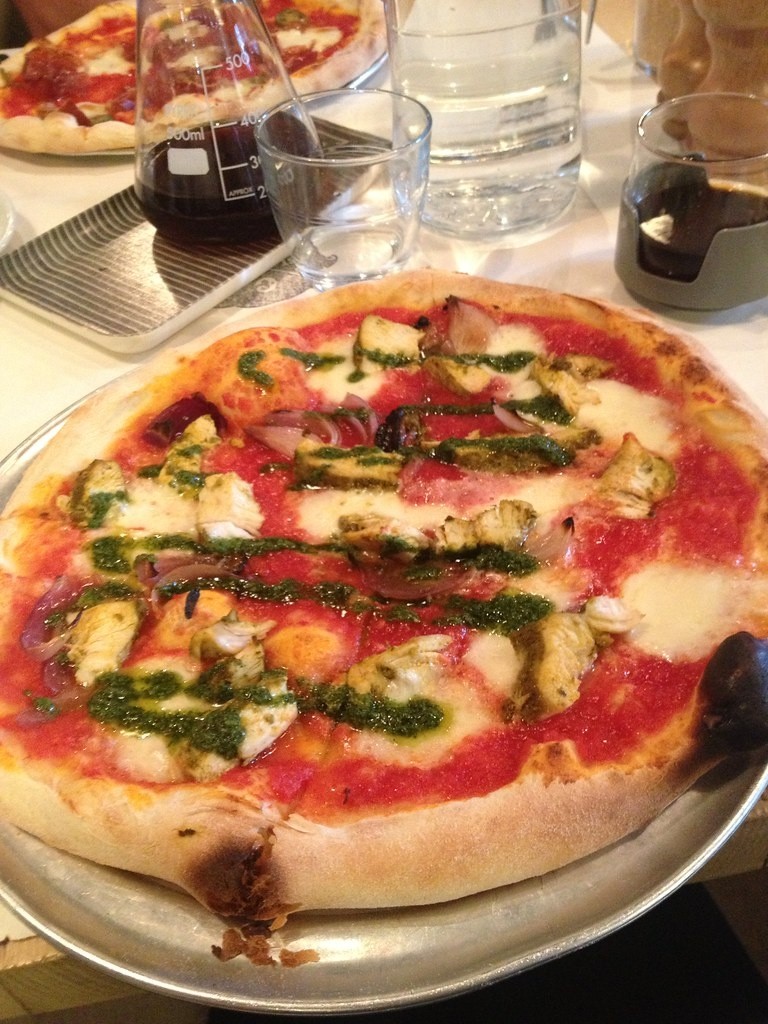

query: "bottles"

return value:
[133, 0, 323, 239]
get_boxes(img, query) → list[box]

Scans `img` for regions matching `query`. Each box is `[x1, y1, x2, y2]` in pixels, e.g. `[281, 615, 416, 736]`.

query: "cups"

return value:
[254, 88, 432, 292]
[632, 0, 682, 81]
[616, 92, 768, 313]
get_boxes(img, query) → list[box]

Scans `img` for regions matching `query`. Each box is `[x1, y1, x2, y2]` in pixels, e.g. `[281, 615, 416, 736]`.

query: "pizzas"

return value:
[0, 0, 389, 156]
[0, 265, 768, 929]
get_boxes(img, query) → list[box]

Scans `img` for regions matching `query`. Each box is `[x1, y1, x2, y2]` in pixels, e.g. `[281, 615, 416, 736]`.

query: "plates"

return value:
[0, 370, 768, 1017]
[37, 48, 391, 158]
[0, 106, 396, 353]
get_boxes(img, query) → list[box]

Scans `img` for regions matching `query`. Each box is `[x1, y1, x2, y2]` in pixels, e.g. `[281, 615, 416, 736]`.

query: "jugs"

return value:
[381, 0, 597, 244]
